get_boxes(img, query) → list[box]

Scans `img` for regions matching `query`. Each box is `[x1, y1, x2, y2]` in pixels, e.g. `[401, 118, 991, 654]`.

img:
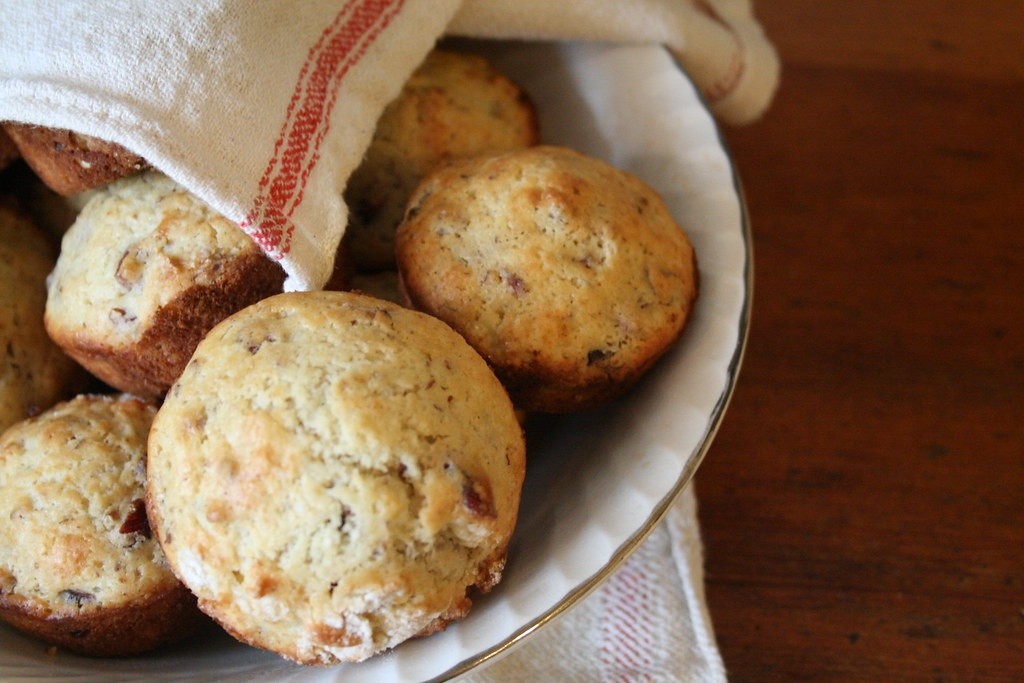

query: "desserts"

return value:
[0, 44, 702, 673]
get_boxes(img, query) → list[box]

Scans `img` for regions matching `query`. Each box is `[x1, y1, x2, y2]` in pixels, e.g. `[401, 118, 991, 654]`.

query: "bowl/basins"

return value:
[0, 41, 755, 683]
